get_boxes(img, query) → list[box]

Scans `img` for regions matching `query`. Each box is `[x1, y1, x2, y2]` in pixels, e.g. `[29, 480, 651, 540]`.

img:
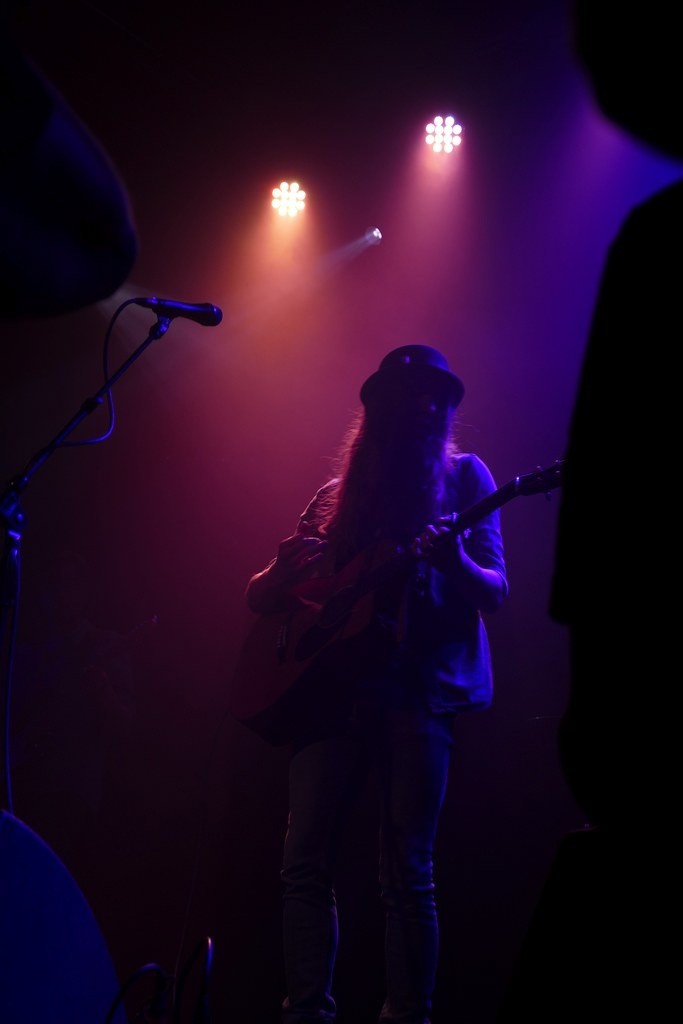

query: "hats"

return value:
[357, 345, 464, 406]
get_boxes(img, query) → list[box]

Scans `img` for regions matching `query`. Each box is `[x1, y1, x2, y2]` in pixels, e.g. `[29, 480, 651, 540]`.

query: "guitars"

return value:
[226, 458, 563, 744]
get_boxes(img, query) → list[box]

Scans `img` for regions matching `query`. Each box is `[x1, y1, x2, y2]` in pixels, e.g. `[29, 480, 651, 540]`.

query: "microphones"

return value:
[134, 297, 223, 326]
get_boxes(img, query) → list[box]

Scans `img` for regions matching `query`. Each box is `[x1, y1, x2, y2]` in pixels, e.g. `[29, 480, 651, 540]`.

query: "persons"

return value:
[545, 3, 682, 1022]
[247, 342, 514, 1022]
